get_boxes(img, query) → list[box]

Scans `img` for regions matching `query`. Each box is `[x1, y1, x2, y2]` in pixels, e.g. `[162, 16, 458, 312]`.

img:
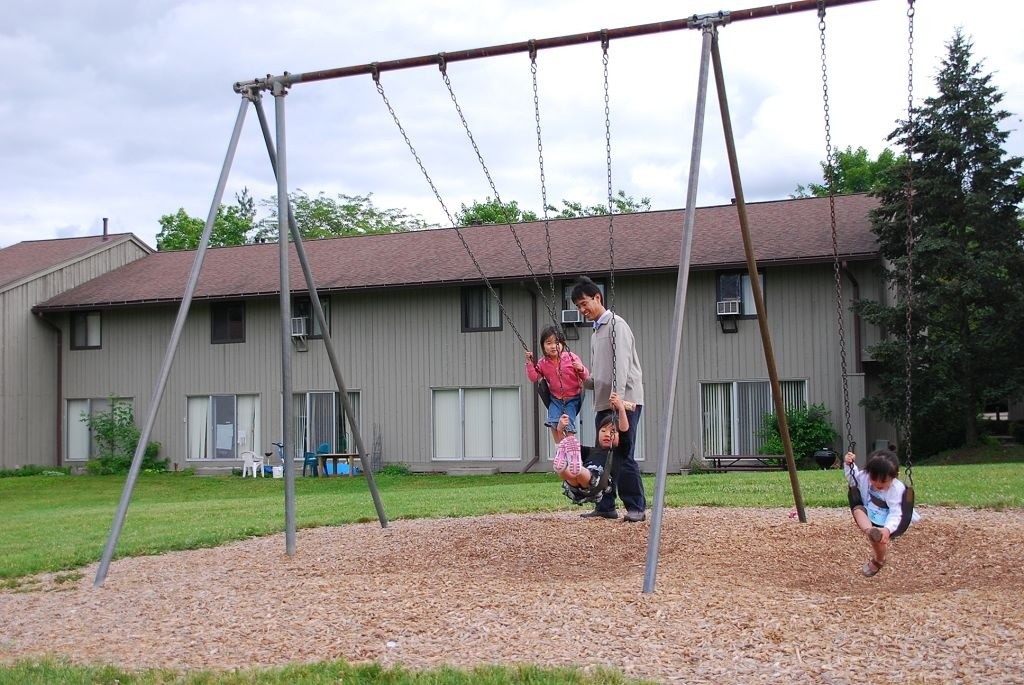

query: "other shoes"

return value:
[579, 509, 619, 520]
[861, 556, 887, 577]
[623, 510, 647, 523]
[868, 527, 882, 543]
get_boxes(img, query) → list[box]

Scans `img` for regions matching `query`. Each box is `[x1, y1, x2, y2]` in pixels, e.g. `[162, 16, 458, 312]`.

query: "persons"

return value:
[522, 324, 589, 444]
[843, 442, 920, 577]
[571, 283, 646, 521]
[551, 392, 629, 505]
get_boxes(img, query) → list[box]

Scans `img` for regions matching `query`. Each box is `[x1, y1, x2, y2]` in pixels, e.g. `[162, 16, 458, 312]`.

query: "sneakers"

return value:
[553, 437, 568, 474]
[564, 436, 582, 476]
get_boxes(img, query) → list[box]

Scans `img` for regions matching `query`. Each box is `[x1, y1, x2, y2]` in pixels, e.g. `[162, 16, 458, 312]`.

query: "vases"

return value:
[680, 468, 692, 476]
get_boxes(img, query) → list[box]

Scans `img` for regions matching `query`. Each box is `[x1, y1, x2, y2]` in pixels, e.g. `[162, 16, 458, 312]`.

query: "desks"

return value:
[315, 453, 370, 478]
[705, 455, 787, 474]
[989, 436, 1015, 449]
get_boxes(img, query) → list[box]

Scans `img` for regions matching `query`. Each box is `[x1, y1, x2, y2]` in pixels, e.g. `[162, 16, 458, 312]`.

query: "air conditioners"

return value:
[291, 317, 308, 336]
[875, 440, 890, 451]
[717, 300, 741, 315]
[562, 310, 582, 322]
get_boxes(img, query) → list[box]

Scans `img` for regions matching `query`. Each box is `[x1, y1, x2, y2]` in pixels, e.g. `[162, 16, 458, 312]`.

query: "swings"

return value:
[370, 49, 588, 420]
[529, 32, 619, 499]
[815, 0, 921, 542]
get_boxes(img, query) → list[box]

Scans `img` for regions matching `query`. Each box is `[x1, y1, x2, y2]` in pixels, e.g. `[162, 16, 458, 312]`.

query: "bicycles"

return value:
[256, 441, 286, 478]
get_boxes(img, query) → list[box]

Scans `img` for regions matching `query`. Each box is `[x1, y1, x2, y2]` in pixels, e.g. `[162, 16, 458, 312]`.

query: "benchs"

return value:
[699, 468, 784, 470]
[1001, 444, 1018, 449]
[717, 465, 788, 468]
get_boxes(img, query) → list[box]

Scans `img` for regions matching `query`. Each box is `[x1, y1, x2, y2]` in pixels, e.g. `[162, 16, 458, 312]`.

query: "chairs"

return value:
[240, 451, 264, 478]
[303, 443, 330, 478]
